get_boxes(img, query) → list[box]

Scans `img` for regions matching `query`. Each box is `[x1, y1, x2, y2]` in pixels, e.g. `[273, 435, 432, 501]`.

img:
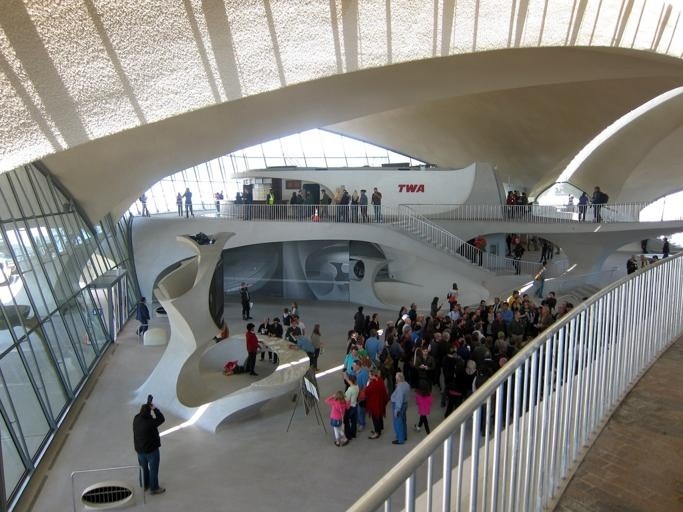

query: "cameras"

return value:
[147, 394, 153, 405]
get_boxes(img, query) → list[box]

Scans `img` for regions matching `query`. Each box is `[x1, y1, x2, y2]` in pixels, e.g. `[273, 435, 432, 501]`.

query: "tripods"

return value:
[286, 377, 327, 433]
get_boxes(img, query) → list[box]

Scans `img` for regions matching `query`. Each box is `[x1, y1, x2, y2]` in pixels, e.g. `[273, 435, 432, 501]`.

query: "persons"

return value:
[139, 193, 150, 216]
[218, 281, 323, 377]
[237, 189, 251, 222]
[290, 187, 382, 223]
[267, 190, 275, 219]
[475, 234, 560, 277]
[136, 297, 151, 338]
[507, 189, 529, 220]
[627, 238, 669, 276]
[217, 190, 223, 212]
[177, 188, 194, 218]
[133, 403, 166, 496]
[324, 285, 619, 446]
[567, 186, 603, 223]
